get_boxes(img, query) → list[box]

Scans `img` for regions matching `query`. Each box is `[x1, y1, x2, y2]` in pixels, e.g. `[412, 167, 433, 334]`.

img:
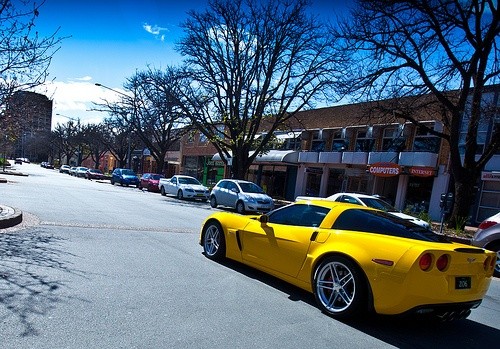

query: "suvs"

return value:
[110, 168, 139, 187]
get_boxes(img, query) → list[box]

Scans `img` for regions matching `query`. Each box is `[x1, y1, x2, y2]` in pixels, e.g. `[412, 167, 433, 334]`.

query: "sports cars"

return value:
[198, 198, 497, 321]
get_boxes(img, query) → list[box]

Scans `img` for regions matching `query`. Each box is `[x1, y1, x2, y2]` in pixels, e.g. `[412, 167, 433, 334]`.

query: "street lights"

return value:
[55, 113, 82, 165]
[94, 82, 134, 167]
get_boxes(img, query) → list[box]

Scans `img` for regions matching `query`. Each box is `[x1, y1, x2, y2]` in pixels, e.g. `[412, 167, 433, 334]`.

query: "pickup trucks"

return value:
[294, 192, 432, 231]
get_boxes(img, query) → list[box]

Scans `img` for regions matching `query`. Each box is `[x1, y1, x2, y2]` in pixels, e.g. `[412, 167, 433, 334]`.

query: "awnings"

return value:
[211, 150, 299, 165]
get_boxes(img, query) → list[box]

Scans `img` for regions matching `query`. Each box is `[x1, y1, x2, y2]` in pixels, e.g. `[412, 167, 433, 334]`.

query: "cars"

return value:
[139, 172, 164, 193]
[69, 166, 77, 176]
[75, 167, 88, 179]
[14, 158, 22, 165]
[41, 161, 54, 169]
[157, 175, 208, 201]
[20, 158, 30, 163]
[209, 178, 275, 214]
[59, 164, 70, 173]
[83, 168, 104, 180]
[472, 206, 500, 277]
